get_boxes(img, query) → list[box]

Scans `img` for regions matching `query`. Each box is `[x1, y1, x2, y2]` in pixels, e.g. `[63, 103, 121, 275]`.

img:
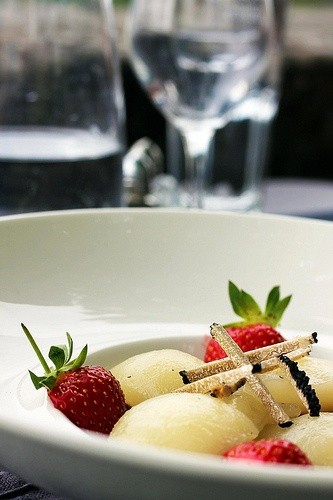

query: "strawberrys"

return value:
[204, 279, 291, 361]
[224, 438, 311, 466]
[21, 323, 126, 434]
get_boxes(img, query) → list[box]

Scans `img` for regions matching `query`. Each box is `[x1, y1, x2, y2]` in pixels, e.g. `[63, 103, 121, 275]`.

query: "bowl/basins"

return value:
[0, 209, 333, 499]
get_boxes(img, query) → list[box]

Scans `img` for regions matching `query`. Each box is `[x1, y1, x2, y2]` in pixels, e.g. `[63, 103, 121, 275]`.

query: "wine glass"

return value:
[123, 0, 274, 208]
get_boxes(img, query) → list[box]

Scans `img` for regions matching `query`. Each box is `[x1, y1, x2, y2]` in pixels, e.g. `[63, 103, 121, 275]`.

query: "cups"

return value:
[0, 0, 123, 216]
